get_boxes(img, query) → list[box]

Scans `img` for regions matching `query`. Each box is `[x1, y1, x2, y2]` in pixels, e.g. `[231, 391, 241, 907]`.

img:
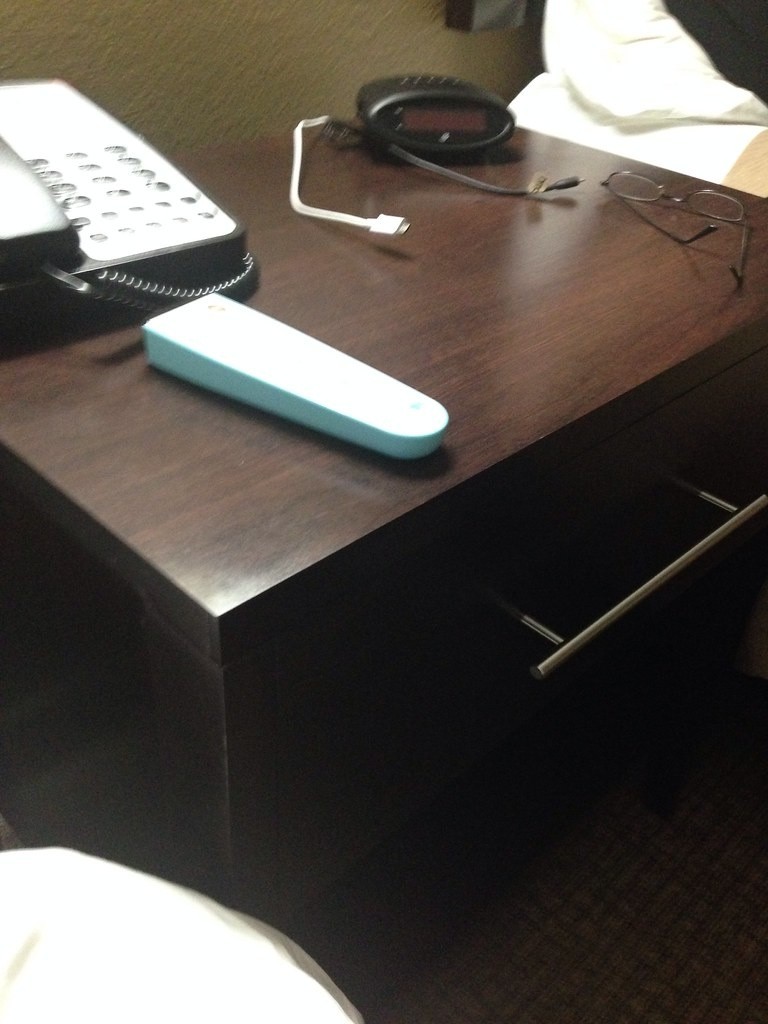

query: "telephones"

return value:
[0, 79, 262, 339]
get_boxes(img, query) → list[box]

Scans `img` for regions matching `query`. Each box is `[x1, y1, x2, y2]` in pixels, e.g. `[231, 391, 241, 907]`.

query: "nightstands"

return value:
[0, 111, 768, 994]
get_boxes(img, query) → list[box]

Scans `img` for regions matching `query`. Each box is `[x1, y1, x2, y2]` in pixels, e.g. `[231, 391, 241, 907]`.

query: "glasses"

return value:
[599, 170, 748, 288]
